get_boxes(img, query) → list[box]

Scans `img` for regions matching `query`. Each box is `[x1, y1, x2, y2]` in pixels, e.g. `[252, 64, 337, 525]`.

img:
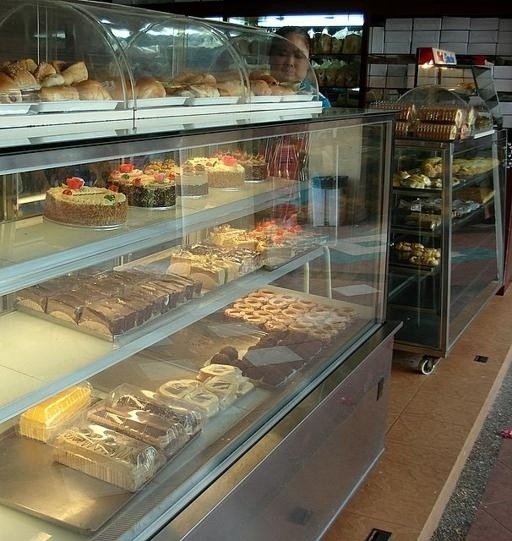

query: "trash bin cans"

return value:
[312, 176, 349, 227]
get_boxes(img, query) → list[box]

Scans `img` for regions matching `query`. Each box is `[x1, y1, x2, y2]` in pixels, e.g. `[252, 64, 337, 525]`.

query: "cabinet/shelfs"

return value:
[0, 2, 403, 539]
[385, 82, 506, 375]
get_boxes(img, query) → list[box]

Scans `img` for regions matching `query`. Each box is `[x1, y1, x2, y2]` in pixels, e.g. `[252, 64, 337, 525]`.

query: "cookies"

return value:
[369, 98, 475, 145]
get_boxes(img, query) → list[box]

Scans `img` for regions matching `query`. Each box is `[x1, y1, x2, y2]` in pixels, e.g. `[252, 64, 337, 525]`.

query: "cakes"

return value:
[107, 150, 269, 208]
[43, 178, 128, 225]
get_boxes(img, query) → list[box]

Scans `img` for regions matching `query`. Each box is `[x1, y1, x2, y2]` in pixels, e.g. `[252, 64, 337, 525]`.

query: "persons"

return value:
[247, 26, 335, 119]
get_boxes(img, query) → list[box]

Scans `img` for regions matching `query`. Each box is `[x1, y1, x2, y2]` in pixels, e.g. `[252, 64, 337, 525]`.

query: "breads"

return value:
[223, 288, 357, 345]
[86, 405, 190, 449]
[111, 392, 203, 438]
[20, 385, 92, 443]
[158, 364, 254, 420]
[210, 331, 322, 384]
[17, 266, 202, 337]
[167, 242, 262, 291]
[207, 215, 330, 267]
[393, 153, 501, 268]
[53, 424, 166, 491]
[0, 53, 294, 101]
